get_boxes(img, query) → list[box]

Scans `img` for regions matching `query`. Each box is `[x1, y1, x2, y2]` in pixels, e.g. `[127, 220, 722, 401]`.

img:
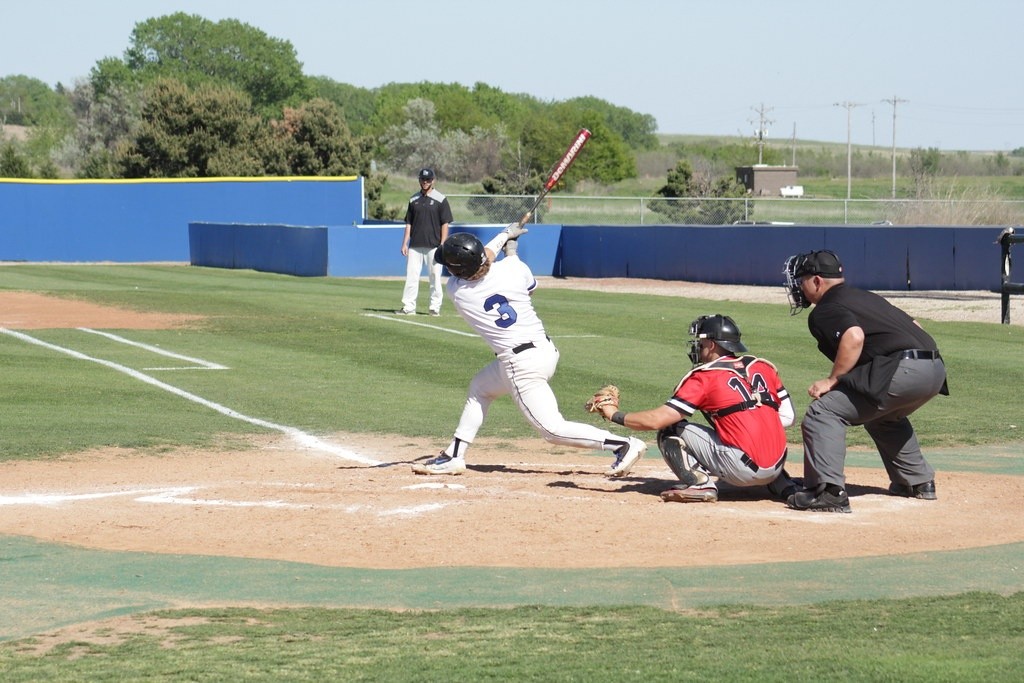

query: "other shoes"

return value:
[660, 488, 717, 502]
[781, 484, 803, 500]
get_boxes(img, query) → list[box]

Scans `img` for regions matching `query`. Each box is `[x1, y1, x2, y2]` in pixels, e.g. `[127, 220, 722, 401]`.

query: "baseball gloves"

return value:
[584, 385, 621, 416]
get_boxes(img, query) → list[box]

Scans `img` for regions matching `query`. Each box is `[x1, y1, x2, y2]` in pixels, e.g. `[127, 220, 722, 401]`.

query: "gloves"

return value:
[501, 239, 518, 256]
[501, 222, 528, 239]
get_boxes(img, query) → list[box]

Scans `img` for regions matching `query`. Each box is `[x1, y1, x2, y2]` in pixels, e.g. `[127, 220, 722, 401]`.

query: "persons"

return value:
[411, 221, 647, 479]
[394, 168, 453, 316]
[585, 313, 804, 503]
[782, 249, 950, 512]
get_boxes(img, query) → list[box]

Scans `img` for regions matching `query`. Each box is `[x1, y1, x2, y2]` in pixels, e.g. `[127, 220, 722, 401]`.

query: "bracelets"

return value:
[611, 411, 626, 426]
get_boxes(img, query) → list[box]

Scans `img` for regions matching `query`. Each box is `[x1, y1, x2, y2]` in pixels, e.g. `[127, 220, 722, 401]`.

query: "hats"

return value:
[793, 250, 843, 278]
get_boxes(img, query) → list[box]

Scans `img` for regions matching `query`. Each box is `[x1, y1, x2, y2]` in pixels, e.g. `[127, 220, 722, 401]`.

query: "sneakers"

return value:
[604, 437, 647, 477]
[788, 491, 852, 512]
[889, 480, 936, 499]
[411, 450, 465, 475]
[429, 310, 438, 316]
[394, 310, 415, 315]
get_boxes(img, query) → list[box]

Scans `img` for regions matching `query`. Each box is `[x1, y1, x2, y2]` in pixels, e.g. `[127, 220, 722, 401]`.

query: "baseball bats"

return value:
[519, 126, 594, 228]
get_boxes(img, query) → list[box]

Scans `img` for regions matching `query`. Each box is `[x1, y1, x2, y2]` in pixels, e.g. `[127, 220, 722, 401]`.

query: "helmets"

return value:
[698, 315, 747, 352]
[434, 233, 487, 280]
[418, 169, 434, 179]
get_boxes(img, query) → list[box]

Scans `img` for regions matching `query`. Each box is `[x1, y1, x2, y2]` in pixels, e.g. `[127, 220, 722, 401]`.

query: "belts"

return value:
[900, 349, 940, 359]
[495, 334, 550, 356]
[741, 447, 787, 473]
